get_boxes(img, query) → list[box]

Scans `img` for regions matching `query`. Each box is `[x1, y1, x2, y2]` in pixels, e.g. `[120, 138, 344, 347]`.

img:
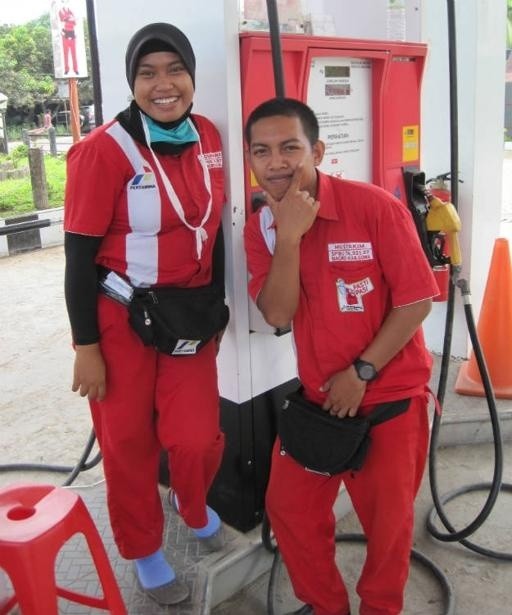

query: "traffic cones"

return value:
[448, 235, 512, 400]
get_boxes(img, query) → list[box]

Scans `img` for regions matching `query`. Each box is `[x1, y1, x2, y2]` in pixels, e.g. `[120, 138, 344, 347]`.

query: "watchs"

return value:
[353, 359, 378, 385]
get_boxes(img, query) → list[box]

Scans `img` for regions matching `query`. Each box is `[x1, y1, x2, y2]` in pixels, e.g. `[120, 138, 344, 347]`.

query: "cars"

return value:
[81, 101, 96, 132]
[50, 110, 85, 128]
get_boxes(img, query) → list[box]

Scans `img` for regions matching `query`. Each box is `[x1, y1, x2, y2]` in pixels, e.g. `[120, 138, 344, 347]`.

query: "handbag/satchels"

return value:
[277, 391, 371, 474]
[128, 284, 229, 355]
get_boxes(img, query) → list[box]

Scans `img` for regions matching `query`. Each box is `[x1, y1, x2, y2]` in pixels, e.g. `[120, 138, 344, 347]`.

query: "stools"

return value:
[0, 483, 129, 615]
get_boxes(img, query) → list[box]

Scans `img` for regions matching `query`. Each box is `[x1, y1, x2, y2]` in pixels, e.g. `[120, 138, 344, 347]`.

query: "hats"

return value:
[126, 23, 195, 91]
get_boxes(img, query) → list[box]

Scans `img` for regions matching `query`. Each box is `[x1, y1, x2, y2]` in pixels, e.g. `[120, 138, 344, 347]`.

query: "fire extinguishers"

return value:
[425, 171, 464, 302]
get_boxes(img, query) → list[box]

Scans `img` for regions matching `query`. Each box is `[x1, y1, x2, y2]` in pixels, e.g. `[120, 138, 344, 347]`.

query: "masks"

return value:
[139, 109, 199, 145]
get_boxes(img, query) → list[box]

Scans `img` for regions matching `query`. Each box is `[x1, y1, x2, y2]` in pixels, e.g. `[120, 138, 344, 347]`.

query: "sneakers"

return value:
[167, 486, 225, 549]
[131, 560, 189, 605]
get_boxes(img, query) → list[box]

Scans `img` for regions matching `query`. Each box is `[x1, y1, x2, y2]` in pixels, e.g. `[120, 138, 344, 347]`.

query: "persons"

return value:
[64, 24, 231, 607]
[59, 1, 80, 74]
[243, 97, 434, 615]
[43, 110, 51, 133]
